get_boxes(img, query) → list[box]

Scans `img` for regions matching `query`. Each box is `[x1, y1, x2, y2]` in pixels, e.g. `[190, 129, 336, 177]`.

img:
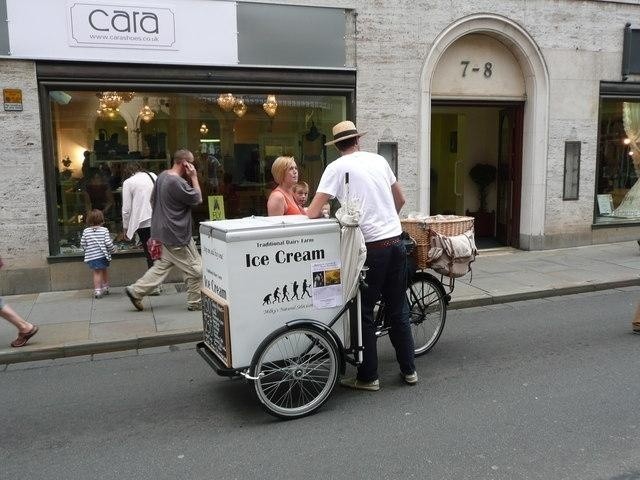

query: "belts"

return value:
[366, 237, 401, 248]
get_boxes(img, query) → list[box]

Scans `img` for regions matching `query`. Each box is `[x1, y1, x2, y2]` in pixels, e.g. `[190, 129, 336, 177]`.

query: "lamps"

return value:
[233, 98, 247, 119]
[139, 96, 155, 125]
[95, 91, 135, 121]
[216, 93, 234, 113]
[263, 93, 279, 120]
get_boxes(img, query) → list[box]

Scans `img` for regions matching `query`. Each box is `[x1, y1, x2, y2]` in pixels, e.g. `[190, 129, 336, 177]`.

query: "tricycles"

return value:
[196, 238, 451, 419]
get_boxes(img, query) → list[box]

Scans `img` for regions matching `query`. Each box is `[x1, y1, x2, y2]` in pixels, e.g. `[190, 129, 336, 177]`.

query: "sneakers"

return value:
[124, 286, 144, 311]
[159, 284, 163, 292]
[149, 286, 161, 295]
[631, 327, 640, 335]
[102, 287, 109, 296]
[400, 371, 419, 384]
[93, 289, 103, 298]
[340, 377, 379, 391]
[187, 301, 202, 312]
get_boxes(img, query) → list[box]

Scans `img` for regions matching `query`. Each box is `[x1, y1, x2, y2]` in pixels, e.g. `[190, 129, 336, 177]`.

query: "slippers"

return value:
[11, 324, 39, 346]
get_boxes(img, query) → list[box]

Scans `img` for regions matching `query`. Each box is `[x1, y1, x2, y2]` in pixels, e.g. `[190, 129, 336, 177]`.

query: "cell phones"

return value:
[183, 161, 189, 167]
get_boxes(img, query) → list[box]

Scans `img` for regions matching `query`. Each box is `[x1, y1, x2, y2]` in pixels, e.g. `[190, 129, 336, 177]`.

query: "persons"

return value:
[267, 156, 305, 217]
[306, 121, 418, 391]
[201, 144, 225, 192]
[294, 180, 308, 205]
[82, 151, 89, 175]
[82, 167, 117, 221]
[125, 149, 203, 311]
[301, 126, 326, 198]
[121, 160, 163, 296]
[80, 208, 118, 298]
[321, 202, 330, 217]
[218, 173, 240, 216]
[0, 299, 39, 347]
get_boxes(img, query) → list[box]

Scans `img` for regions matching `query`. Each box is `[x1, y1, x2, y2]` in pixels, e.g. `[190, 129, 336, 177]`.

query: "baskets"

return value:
[400, 213, 475, 268]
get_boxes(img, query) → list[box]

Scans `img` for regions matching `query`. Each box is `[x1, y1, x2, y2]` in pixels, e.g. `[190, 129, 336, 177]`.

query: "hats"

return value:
[324, 121, 368, 147]
